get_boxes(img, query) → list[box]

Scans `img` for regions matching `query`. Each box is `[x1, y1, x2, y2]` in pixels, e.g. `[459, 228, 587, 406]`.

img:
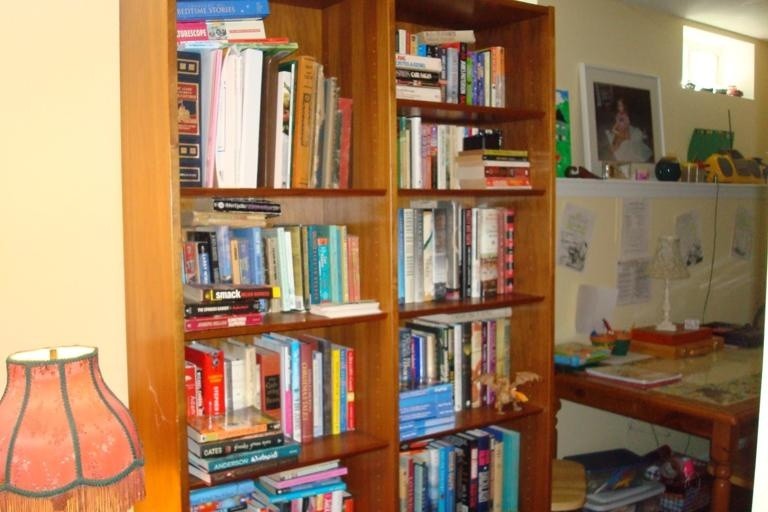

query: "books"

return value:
[586, 361, 684, 391]
[184, 332, 355, 487]
[179, 195, 381, 331]
[397, 199, 515, 303]
[175, 0, 354, 189]
[189, 460, 354, 512]
[397, 307, 512, 441]
[396, 115, 532, 189]
[394, 29, 505, 108]
[398, 426, 520, 511]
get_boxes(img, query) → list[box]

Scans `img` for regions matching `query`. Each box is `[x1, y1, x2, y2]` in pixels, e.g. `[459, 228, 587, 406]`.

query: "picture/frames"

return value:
[575, 61, 668, 177]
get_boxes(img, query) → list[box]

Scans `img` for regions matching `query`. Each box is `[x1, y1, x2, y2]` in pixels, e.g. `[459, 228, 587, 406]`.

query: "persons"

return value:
[612, 100, 630, 151]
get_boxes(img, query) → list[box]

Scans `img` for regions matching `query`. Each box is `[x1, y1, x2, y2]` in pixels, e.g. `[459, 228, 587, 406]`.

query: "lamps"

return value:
[0, 343, 148, 512]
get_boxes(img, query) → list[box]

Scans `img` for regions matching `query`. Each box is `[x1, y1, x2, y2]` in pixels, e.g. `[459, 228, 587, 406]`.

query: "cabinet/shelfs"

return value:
[117, 0, 395, 512]
[395, 0, 556, 512]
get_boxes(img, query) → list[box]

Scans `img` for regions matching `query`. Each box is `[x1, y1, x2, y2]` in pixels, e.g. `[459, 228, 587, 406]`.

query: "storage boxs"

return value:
[560, 442, 668, 511]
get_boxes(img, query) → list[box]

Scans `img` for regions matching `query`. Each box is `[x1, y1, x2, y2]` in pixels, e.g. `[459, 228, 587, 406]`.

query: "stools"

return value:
[706, 442, 758, 511]
[549, 455, 589, 511]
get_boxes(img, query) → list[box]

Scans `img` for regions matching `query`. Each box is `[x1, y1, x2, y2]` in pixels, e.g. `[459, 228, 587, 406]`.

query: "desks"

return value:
[550, 321, 765, 511]
